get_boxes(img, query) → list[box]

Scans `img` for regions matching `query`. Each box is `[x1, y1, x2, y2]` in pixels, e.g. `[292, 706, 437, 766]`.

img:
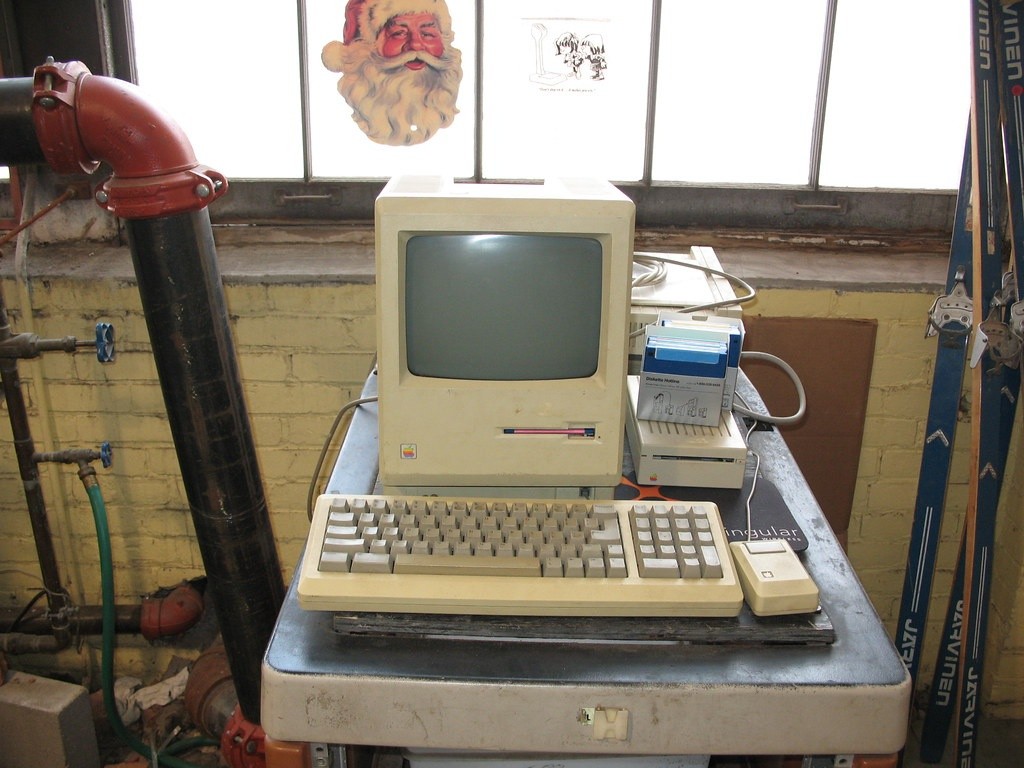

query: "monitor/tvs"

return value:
[375, 172, 637, 485]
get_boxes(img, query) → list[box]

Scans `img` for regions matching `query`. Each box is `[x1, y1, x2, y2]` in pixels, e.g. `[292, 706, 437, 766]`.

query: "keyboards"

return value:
[296, 494, 744, 618]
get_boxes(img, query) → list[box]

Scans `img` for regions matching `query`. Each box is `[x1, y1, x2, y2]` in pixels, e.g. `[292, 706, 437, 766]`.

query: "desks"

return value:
[259, 361, 910, 768]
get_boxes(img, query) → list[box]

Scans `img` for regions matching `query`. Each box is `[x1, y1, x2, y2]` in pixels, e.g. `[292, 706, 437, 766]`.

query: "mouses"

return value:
[730, 540, 819, 617]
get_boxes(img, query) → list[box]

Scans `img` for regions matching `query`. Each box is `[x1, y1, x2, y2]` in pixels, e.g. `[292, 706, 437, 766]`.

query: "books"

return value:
[636, 310, 745, 427]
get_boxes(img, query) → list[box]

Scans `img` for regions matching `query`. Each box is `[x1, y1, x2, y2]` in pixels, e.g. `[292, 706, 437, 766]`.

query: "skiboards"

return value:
[894, 1, 1024, 768]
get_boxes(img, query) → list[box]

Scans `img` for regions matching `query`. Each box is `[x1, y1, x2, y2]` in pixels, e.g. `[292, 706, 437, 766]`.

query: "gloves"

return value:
[131, 667, 190, 710]
